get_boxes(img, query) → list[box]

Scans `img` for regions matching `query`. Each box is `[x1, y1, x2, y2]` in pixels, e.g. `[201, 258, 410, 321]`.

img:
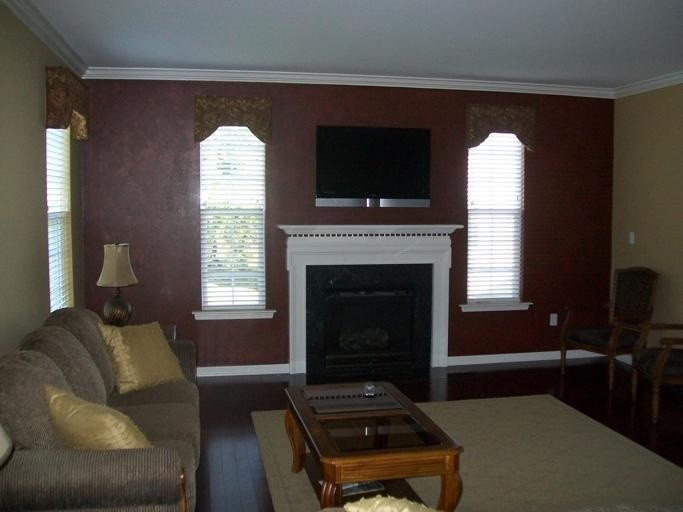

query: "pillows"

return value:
[41, 382, 152, 451]
[95, 322, 186, 395]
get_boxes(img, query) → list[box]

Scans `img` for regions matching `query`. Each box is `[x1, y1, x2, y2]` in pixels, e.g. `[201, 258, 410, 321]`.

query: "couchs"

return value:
[0, 307, 203, 512]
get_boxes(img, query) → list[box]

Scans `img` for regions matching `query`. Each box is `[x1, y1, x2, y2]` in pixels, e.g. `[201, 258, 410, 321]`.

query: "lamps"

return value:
[96, 243, 139, 326]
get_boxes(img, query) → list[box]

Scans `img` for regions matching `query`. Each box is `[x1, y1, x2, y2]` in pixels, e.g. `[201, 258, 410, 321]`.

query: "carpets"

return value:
[250, 394, 682, 512]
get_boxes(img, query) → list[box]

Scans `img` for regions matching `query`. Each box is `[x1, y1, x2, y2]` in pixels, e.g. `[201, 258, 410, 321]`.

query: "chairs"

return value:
[561, 266, 659, 392]
[630, 323, 683, 423]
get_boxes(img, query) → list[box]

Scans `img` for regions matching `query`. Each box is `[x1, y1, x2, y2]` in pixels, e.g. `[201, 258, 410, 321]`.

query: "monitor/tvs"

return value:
[315, 125, 431, 210]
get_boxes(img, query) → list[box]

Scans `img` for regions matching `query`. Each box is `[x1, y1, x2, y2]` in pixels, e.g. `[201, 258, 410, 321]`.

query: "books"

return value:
[341, 481, 386, 498]
[318, 478, 379, 491]
